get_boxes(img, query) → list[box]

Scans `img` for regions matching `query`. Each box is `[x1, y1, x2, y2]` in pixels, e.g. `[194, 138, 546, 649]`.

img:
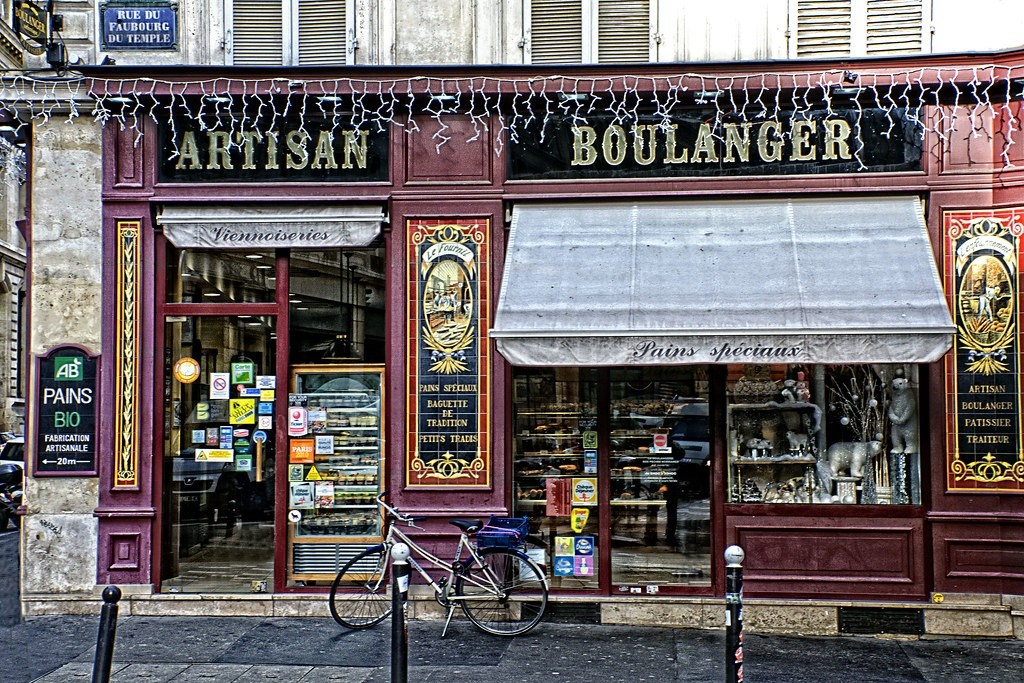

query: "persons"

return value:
[639, 423, 685, 547]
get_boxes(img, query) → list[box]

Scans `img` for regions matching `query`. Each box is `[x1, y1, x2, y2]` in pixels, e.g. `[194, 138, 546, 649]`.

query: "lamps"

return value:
[46, 41, 64, 71]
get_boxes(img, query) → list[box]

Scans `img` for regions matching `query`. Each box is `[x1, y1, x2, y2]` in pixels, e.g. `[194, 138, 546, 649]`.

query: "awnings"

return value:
[158, 202, 388, 254]
[488, 192, 959, 367]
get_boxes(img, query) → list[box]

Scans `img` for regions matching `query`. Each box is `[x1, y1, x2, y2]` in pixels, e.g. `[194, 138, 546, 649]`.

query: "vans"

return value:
[164, 399, 275, 519]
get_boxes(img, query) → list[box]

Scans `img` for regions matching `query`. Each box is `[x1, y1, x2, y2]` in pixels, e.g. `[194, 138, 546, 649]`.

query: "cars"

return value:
[0, 429, 26, 531]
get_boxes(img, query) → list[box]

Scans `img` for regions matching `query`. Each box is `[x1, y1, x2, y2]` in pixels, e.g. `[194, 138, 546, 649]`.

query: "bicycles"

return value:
[326, 490, 553, 638]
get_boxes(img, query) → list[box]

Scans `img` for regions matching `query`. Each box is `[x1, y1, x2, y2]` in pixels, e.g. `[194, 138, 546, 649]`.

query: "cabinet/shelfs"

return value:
[509, 404, 671, 547]
[290, 364, 387, 542]
